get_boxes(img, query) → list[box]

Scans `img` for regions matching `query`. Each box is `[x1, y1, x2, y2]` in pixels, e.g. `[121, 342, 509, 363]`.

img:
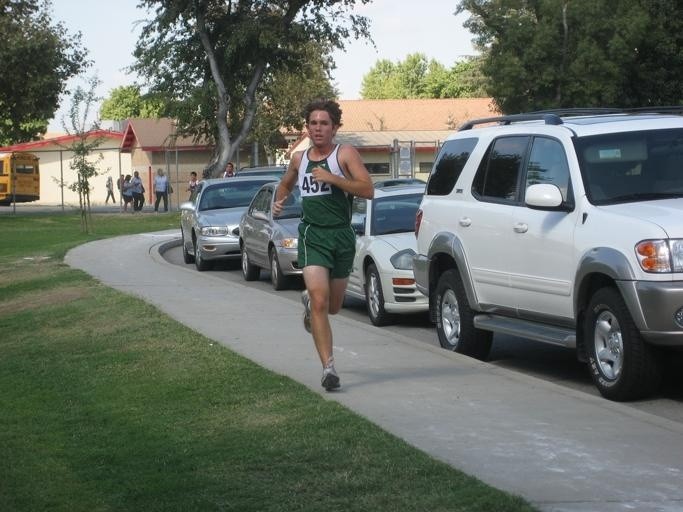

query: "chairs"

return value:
[538, 142, 627, 203]
[202, 187, 225, 209]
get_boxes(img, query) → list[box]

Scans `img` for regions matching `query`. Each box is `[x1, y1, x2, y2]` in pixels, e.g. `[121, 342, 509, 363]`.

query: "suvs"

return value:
[414, 107, 681, 403]
[235, 163, 286, 176]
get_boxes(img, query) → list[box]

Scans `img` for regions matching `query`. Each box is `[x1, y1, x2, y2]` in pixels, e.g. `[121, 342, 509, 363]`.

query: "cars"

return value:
[238, 184, 305, 291]
[179, 176, 279, 270]
[345, 184, 433, 326]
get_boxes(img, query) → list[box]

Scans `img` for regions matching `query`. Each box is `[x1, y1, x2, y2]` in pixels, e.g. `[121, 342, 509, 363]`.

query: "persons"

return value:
[122, 175, 136, 213]
[130, 171, 145, 213]
[153, 167, 170, 215]
[104, 174, 116, 204]
[117, 174, 125, 204]
[185, 171, 199, 193]
[222, 162, 236, 177]
[270, 100, 375, 391]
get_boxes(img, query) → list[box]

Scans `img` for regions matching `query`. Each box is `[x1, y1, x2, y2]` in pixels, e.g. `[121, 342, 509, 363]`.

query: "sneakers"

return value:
[321, 368, 340, 391]
[301, 290, 313, 332]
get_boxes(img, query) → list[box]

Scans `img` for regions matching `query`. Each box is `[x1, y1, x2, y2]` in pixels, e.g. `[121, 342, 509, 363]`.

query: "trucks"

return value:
[0, 151, 40, 203]
